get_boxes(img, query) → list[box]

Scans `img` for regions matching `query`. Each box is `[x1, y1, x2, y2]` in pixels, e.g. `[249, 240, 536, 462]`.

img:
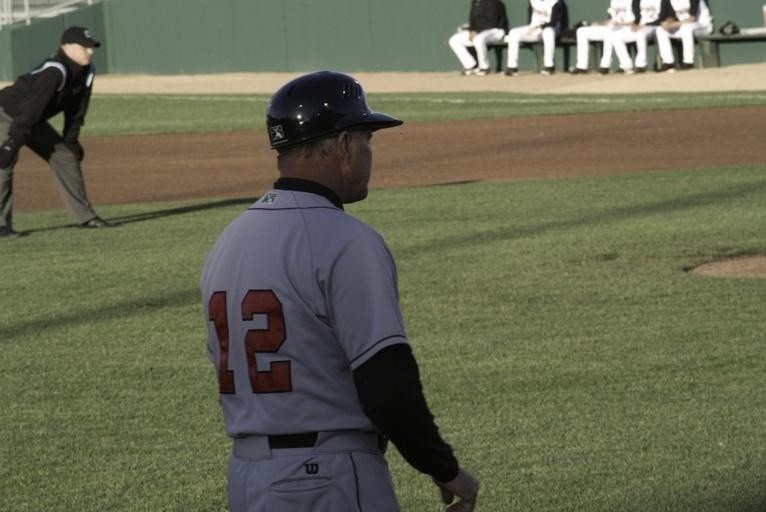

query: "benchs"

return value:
[464, 26, 766, 70]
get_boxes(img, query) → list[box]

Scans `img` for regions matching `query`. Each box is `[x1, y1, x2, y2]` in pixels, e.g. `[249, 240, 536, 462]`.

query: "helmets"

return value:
[267, 71, 403, 149]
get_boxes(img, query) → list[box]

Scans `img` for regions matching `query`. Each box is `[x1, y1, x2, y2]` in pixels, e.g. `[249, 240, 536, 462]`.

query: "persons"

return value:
[0, 26, 110, 239]
[569, 0, 715, 75]
[448, 0, 568, 77]
[200, 70, 480, 512]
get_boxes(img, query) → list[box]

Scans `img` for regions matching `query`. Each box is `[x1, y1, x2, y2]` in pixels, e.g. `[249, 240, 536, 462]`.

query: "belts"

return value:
[269, 432, 319, 450]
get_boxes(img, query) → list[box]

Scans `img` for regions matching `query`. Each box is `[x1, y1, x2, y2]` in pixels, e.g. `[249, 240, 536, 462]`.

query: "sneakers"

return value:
[84, 216, 109, 227]
[463, 63, 694, 76]
[0, 227, 22, 239]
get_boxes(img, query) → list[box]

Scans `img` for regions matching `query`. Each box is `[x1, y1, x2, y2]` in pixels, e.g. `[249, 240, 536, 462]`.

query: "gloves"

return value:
[0, 139, 22, 169]
[62, 135, 84, 161]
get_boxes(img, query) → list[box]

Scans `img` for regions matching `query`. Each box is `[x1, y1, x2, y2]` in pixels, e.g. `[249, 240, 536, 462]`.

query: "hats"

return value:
[61, 26, 101, 48]
